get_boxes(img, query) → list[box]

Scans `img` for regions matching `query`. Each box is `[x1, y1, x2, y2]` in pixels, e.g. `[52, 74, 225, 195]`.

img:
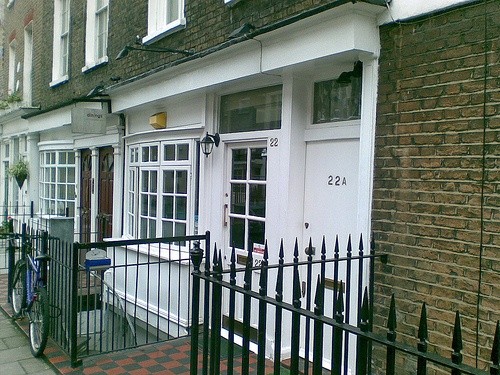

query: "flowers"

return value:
[5, 159, 30, 181]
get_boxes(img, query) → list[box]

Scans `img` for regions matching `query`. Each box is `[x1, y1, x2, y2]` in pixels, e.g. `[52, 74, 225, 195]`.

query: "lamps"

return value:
[115, 46, 136, 60]
[336, 60, 362, 83]
[200, 131, 220, 158]
[227, 22, 255, 39]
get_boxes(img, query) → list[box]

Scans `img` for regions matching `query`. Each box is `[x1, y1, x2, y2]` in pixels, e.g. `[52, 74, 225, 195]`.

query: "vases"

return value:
[15, 174, 27, 190]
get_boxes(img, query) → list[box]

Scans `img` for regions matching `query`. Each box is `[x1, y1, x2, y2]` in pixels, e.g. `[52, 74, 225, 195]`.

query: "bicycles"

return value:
[0, 232, 61, 359]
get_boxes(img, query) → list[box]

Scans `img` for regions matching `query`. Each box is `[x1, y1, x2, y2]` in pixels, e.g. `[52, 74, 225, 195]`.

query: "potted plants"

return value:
[0, 91, 23, 116]
